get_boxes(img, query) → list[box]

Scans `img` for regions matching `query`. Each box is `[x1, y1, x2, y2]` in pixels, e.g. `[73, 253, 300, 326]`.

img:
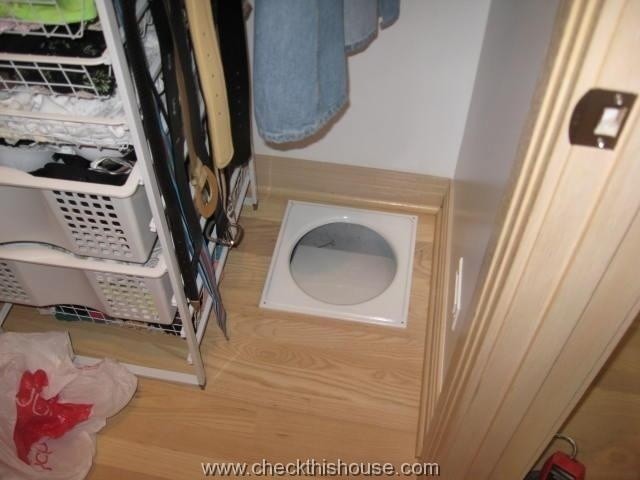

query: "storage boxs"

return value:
[0, 143, 157, 264]
[0, 245, 177, 325]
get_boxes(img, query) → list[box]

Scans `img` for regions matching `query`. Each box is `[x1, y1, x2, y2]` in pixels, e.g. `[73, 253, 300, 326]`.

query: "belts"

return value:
[115, 0, 251, 337]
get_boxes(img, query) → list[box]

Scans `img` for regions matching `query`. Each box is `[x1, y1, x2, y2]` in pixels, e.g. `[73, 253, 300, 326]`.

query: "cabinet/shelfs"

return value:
[0, 0, 257, 390]
